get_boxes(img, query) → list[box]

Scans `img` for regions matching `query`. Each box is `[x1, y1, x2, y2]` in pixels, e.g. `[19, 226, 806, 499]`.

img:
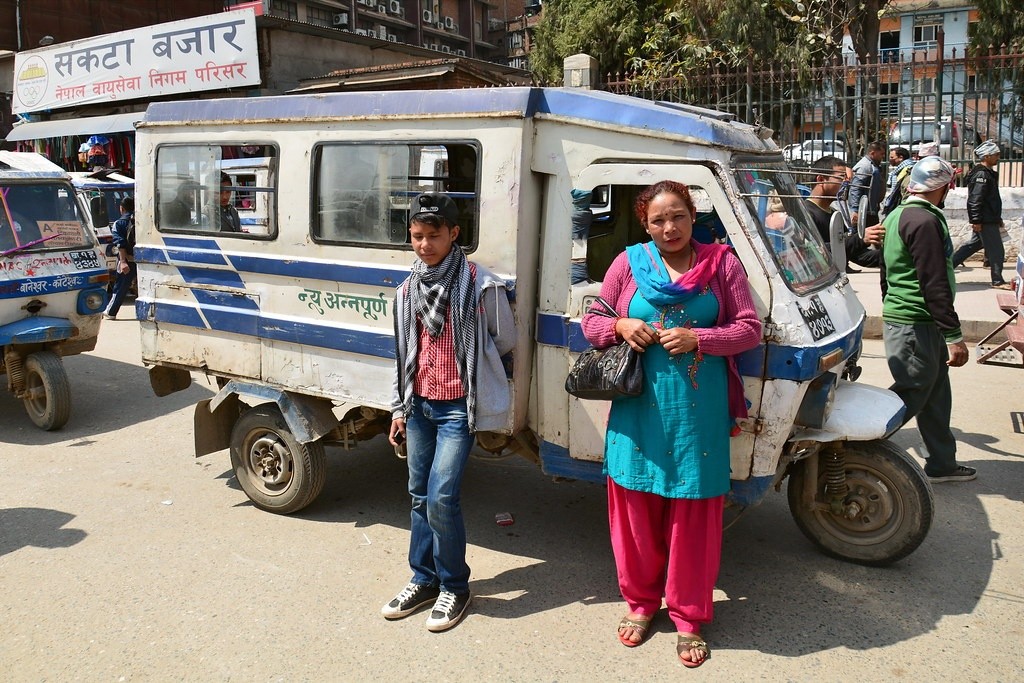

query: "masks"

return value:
[889, 157, 899, 166]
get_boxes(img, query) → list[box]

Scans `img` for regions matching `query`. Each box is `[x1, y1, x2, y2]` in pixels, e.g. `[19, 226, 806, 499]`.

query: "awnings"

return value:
[5, 111, 146, 142]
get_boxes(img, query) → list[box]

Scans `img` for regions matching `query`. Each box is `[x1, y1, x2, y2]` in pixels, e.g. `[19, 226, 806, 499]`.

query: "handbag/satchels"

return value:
[565, 296, 644, 400]
[881, 166, 913, 215]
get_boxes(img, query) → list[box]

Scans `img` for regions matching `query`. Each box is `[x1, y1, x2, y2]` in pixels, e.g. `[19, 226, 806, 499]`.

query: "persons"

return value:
[158, 179, 201, 228]
[846, 139, 887, 274]
[102, 196, 136, 320]
[581, 181, 761, 668]
[952, 139, 1013, 290]
[192, 170, 249, 233]
[803, 156, 886, 270]
[882, 155, 978, 484]
[881, 142, 947, 224]
[380, 195, 516, 632]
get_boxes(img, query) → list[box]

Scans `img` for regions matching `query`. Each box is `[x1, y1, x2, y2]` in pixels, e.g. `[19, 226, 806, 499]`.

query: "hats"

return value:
[974, 141, 1000, 158]
[409, 193, 458, 223]
[918, 142, 938, 156]
[908, 156, 956, 193]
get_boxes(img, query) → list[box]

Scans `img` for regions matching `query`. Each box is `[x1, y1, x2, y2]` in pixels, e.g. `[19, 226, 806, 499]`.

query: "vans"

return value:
[888, 116, 982, 187]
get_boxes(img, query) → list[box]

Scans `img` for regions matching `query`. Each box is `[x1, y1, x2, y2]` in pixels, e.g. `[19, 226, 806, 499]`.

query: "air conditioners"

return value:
[333, 0, 465, 56]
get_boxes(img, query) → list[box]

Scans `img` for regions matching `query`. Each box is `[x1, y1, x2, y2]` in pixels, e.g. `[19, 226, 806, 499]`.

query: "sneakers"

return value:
[382, 583, 440, 618]
[426, 587, 471, 630]
[924, 464, 977, 483]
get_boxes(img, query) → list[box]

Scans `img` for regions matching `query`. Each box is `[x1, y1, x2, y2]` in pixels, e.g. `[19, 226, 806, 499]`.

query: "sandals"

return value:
[618, 612, 651, 647]
[676, 631, 708, 667]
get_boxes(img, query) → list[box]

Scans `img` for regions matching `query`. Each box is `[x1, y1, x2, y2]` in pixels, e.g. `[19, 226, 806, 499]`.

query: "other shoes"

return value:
[103, 311, 116, 319]
[991, 283, 1011, 289]
[847, 266, 861, 273]
[983, 261, 991, 269]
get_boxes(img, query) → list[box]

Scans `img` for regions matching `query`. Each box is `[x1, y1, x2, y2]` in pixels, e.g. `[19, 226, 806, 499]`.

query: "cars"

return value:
[57, 169, 136, 299]
[162, 156, 275, 236]
[782, 139, 849, 165]
[133, 84, 935, 566]
[0, 149, 111, 432]
